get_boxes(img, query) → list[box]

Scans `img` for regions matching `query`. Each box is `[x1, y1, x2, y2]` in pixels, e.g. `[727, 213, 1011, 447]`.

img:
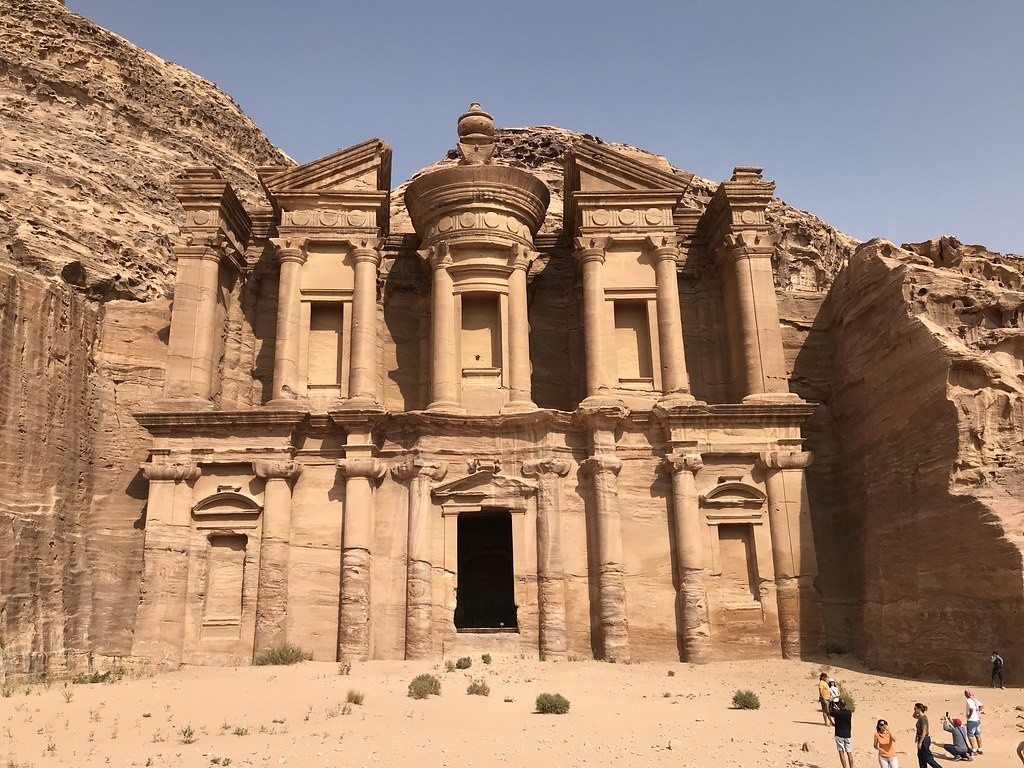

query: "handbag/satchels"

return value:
[968, 748, 972, 756]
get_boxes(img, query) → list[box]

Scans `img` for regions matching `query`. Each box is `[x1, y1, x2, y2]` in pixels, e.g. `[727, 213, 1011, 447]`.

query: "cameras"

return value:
[830, 701, 834, 709]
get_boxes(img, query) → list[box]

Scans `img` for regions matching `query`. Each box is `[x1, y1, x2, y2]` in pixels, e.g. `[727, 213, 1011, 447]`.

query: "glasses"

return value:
[914, 708, 916, 710]
[879, 724, 883, 726]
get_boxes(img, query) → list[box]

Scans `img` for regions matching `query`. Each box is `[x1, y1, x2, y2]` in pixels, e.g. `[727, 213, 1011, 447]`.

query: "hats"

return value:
[829, 678, 835, 682]
[953, 719, 961, 726]
[964, 689, 973, 697]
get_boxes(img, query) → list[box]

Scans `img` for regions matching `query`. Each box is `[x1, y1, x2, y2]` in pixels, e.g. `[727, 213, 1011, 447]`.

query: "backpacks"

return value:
[994, 656, 1002, 668]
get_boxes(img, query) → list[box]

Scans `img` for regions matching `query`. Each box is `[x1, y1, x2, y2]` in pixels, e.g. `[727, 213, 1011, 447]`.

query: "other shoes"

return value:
[977, 748, 983, 754]
[951, 755, 962, 762]
[966, 753, 973, 761]
[972, 749, 977, 755]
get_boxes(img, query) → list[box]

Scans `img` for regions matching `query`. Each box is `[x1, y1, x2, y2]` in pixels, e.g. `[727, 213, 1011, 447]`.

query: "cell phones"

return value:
[946, 712, 948, 717]
[885, 722, 887, 725]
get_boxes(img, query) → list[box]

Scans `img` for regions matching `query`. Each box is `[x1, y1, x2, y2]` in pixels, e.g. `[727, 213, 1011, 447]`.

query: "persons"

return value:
[829, 699, 853, 768]
[828, 678, 840, 717]
[913, 703, 942, 768]
[874, 719, 898, 768]
[990, 651, 1003, 688]
[819, 673, 834, 726]
[943, 717, 973, 761]
[965, 689, 983, 755]
